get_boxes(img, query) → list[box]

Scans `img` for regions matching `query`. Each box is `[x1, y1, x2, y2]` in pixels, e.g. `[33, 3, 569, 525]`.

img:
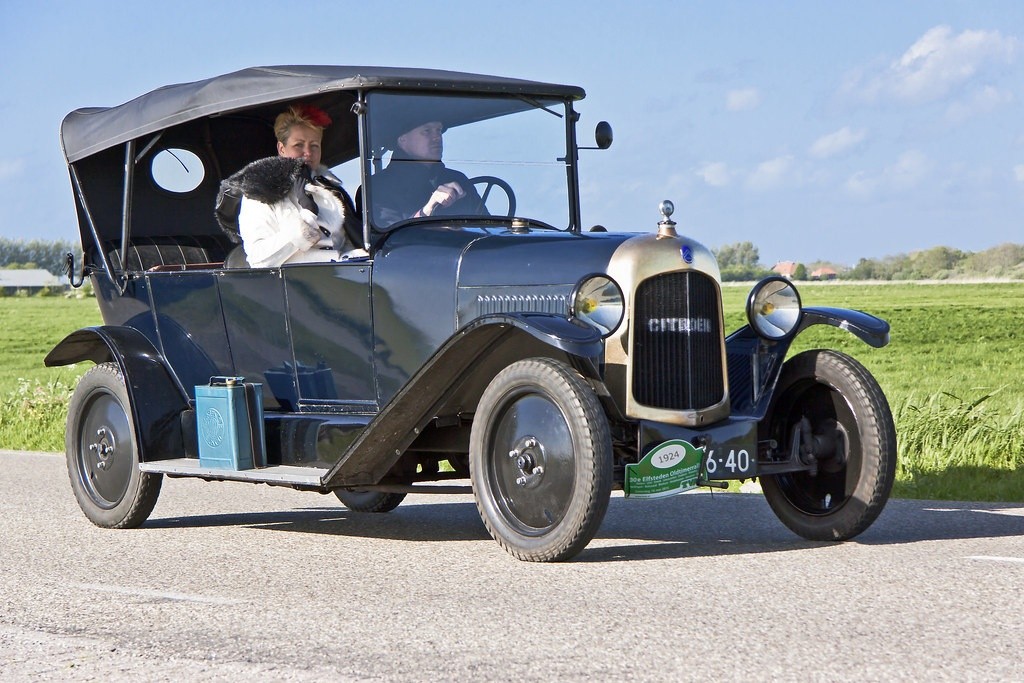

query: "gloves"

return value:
[294, 177, 319, 216]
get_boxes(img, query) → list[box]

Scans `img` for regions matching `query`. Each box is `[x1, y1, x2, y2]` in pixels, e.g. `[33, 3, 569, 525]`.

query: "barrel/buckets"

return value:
[194, 373, 268, 472]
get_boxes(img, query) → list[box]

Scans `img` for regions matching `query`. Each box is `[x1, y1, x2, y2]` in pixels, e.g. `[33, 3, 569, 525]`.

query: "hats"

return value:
[382, 107, 450, 151]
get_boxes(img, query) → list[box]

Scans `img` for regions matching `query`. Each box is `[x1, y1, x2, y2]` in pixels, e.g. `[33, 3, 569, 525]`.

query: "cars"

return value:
[43, 59, 899, 566]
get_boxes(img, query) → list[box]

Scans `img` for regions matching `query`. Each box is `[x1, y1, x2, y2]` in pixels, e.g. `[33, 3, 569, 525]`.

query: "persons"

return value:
[354, 103, 492, 244]
[235, 103, 369, 268]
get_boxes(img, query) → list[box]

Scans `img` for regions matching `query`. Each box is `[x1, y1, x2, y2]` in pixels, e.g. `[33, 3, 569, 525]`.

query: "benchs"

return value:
[89, 234, 238, 272]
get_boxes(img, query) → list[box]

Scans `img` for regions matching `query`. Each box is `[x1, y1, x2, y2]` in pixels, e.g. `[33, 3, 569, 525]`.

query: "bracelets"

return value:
[419, 207, 428, 218]
